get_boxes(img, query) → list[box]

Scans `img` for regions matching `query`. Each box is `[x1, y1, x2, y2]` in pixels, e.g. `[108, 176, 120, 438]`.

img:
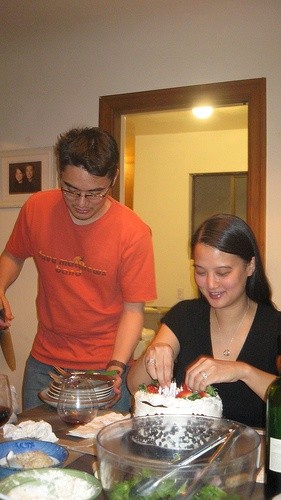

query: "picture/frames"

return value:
[0, 146, 55, 208]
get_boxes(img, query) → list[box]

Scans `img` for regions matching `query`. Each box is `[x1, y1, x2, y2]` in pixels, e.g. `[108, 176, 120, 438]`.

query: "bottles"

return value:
[263, 357, 281, 500]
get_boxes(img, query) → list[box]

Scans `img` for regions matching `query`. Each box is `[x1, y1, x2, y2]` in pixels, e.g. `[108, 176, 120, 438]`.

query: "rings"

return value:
[147, 358, 155, 364]
[200, 371, 207, 379]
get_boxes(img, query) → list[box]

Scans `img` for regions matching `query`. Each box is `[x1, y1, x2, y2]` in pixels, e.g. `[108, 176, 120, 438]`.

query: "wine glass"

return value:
[0, 374, 14, 443]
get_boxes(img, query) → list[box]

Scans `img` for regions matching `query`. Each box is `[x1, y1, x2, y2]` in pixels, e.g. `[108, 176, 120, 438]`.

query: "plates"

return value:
[37, 373, 116, 410]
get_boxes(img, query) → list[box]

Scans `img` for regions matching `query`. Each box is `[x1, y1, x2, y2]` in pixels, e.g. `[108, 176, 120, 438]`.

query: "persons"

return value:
[12, 164, 40, 193]
[126, 214, 281, 426]
[0, 127, 157, 415]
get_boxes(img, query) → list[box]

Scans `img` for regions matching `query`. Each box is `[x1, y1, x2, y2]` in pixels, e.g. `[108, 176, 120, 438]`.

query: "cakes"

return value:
[134, 378, 223, 441]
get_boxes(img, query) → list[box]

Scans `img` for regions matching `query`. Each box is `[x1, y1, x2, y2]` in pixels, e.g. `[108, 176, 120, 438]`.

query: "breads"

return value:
[16, 451, 53, 468]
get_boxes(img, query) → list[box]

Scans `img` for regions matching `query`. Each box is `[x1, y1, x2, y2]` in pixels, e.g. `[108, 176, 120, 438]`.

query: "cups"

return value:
[57, 374, 98, 426]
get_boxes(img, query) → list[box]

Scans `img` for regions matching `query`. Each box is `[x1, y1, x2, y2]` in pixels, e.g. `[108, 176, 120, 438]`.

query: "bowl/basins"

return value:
[0, 439, 69, 480]
[0, 467, 102, 500]
[94, 413, 261, 500]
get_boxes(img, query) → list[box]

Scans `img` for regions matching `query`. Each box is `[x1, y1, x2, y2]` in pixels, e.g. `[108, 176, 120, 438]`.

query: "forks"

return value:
[53, 365, 70, 377]
[47, 370, 65, 384]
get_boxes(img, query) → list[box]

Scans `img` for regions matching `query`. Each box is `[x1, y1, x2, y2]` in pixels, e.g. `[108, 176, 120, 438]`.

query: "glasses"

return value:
[60, 176, 112, 200]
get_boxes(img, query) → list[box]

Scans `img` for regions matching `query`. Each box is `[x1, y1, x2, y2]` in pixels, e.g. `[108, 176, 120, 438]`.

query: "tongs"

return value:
[130, 421, 241, 500]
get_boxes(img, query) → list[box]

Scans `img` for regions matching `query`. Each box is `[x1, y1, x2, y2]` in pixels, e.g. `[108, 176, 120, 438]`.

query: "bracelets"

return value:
[106, 360, 127, 374]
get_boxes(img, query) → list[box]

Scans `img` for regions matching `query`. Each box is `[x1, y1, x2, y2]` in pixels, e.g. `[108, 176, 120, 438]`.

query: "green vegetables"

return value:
[109, 468, 240, 500]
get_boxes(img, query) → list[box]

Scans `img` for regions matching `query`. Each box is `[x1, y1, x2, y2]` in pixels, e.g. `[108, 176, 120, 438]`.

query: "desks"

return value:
[0, 404, 264, 500]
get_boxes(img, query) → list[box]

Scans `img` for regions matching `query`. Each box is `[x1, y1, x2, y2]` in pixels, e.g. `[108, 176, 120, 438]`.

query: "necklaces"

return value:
[214, 296, 248, 357]
